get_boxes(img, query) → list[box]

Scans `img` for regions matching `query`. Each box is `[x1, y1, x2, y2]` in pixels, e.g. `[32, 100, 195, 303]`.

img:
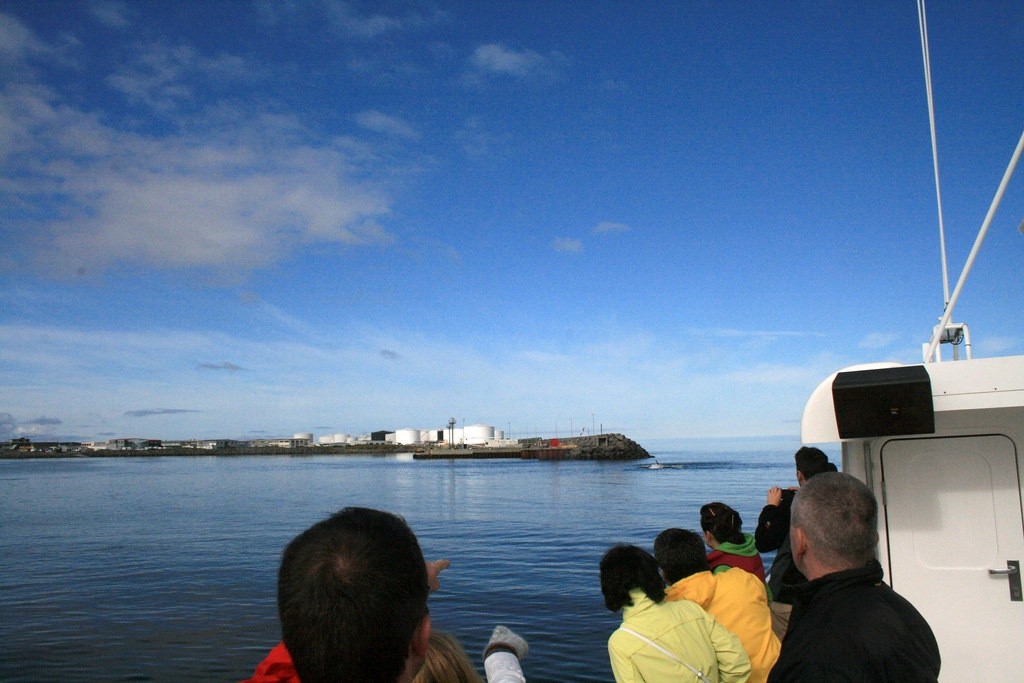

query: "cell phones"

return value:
[781, 488, 793, 499]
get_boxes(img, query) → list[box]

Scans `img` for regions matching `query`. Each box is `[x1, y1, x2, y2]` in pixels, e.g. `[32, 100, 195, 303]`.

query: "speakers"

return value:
[831, 365, 936, 439]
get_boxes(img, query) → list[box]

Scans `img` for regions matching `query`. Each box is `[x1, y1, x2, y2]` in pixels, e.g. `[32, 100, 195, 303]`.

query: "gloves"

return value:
[484, 625, 528, 658]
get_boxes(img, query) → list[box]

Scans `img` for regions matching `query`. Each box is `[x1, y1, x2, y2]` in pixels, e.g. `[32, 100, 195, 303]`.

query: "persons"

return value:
[240, 508, 528, 683]
[700, 502, 770, 604]
[766, 473, 941, 683]
[600, 543, 751, 683]
[653, 528, 781, 683]
[754, 446, 838, 643]
